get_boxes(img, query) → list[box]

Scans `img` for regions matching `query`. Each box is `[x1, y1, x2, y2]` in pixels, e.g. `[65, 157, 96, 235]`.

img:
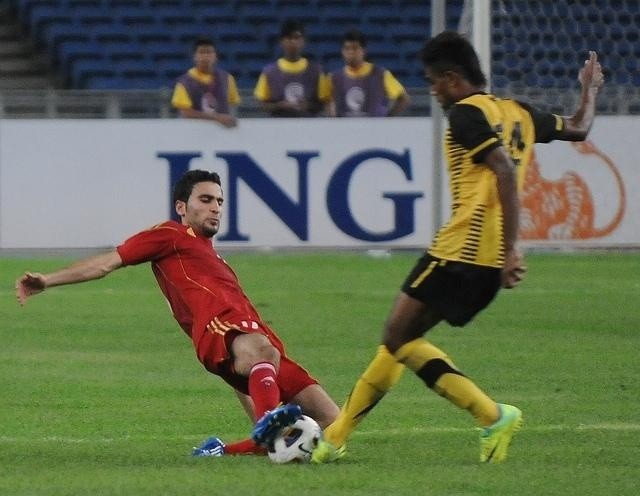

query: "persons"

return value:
[324, 31, 413, 116]
[171, 39, 242, 127]
[308, 29, 605, 466]
[14, 170, 342, 457]
[254, 22, 329, 117]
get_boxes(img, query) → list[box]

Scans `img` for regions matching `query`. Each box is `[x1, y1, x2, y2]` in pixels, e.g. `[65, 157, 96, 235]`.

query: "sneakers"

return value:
[189, 437, 226, 457]
[252, 402, 303, 445]
[477, 402, 523, 463]
[309, 434, 348, 465]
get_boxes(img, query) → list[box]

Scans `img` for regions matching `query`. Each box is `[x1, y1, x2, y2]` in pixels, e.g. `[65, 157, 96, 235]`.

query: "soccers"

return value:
[268, 414, 323, 464]
